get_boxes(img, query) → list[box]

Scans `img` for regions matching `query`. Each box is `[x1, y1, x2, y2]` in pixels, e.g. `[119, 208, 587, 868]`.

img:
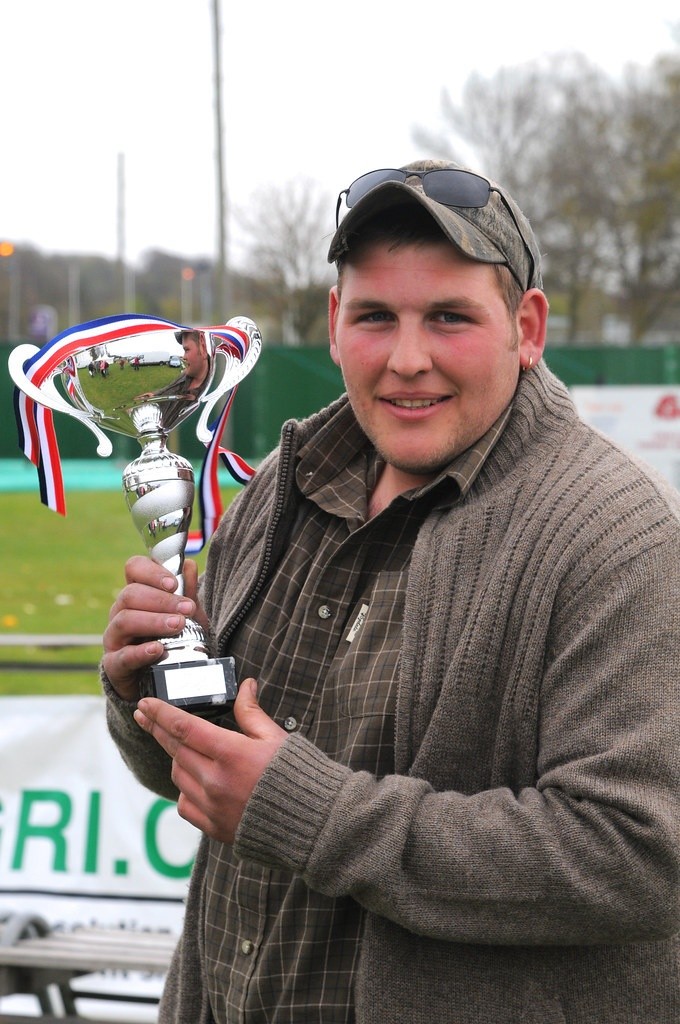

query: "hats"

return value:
[328, 159, 542, 292]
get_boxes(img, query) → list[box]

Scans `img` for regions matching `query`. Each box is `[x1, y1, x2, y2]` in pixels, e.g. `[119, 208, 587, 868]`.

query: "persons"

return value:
[73, 328, 212, 447]
[97, 158, 679, 1024]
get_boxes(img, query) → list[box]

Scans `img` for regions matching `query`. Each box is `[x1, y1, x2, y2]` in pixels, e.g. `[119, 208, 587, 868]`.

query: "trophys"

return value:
[4, 313, 264, 722]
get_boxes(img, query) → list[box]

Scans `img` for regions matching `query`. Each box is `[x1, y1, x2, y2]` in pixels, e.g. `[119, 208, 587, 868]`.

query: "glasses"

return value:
[334, 169, 535, 291]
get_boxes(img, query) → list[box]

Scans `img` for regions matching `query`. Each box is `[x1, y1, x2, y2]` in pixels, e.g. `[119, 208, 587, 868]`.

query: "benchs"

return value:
[0, 907, 179, 1024]
